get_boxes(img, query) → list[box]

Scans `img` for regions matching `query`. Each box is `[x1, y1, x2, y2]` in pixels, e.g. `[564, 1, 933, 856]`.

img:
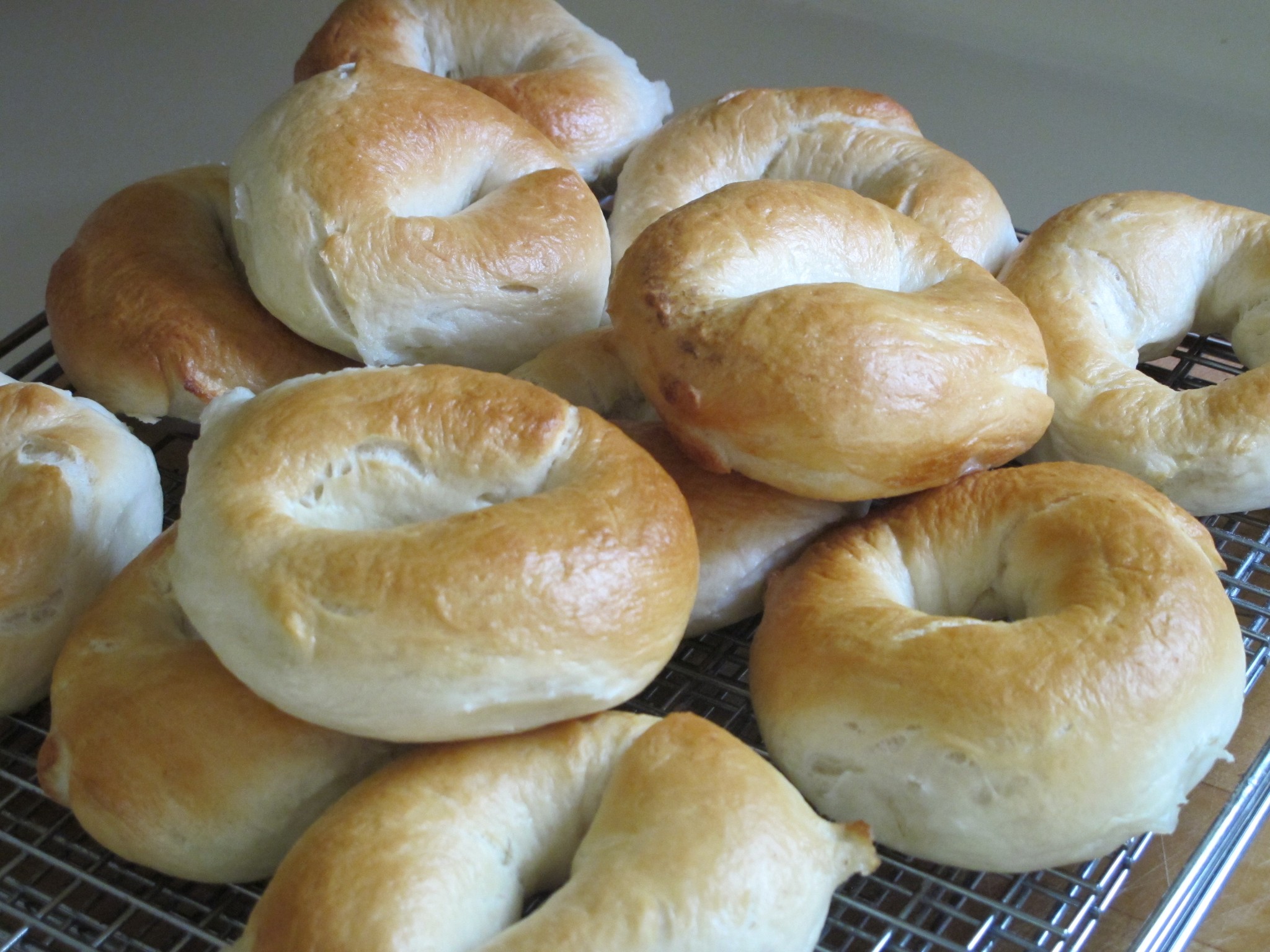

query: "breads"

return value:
[170, 328, 699, 756]
[741, 456, 1251, 880]
[290, 2, 676, 205]
[499, 314, 871, 633]
[596, 79, 1017, 335]
[196, 711, 884, 952]
[600, 177, 1056, 501]
[47, 506, 401, 889]
[43, 160, 362, 429]
[0, 364, 170, 721]
[230, 59, 613, 380]
[986, 186, 1270, 516]
